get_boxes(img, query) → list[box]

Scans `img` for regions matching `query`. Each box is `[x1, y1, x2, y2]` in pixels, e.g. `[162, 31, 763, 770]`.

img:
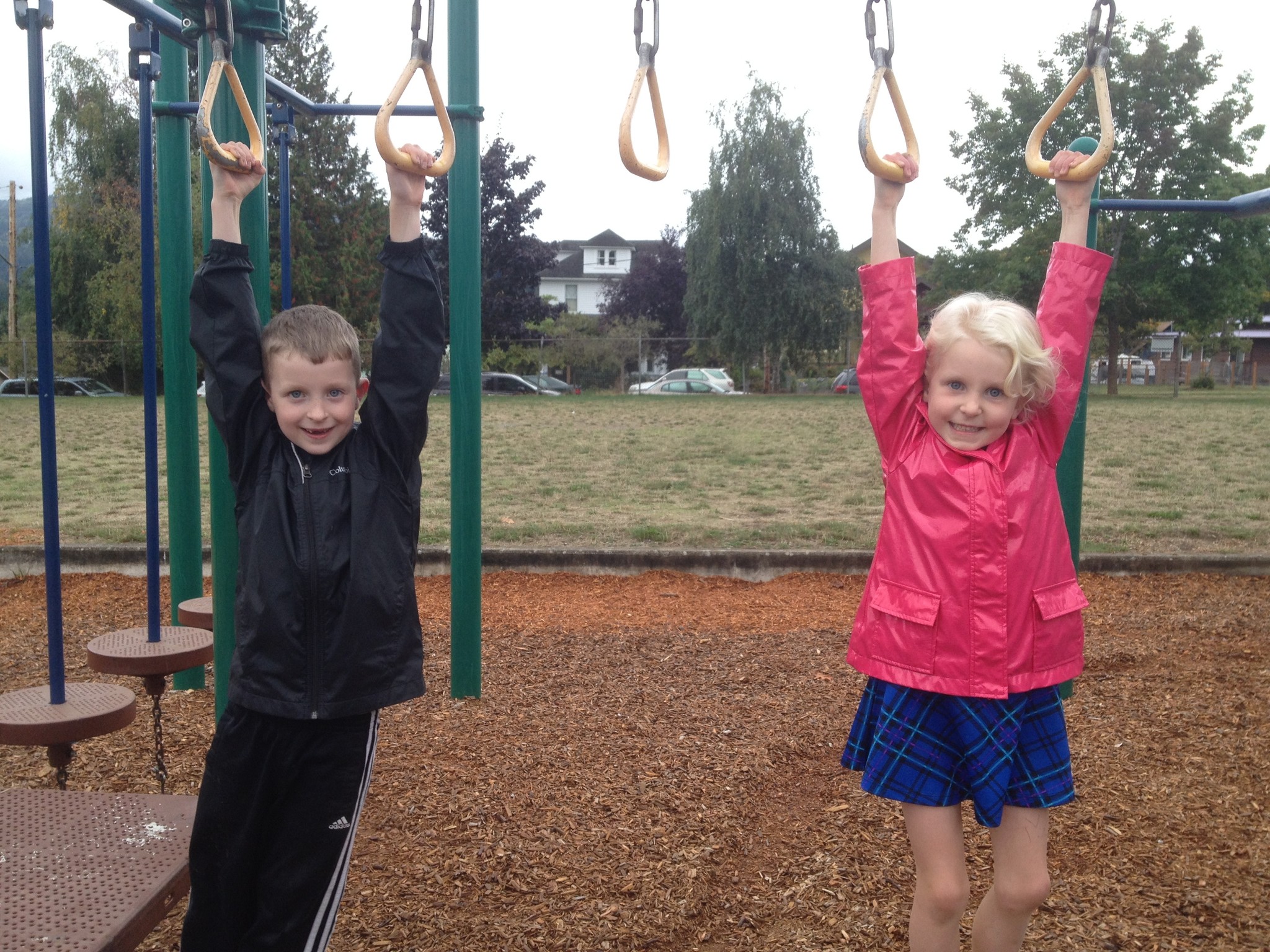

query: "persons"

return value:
[839, 149, 1115, 951]
[181, 141, 446, 952]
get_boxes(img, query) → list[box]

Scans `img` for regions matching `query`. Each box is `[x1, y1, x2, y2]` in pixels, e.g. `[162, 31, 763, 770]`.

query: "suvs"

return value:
[0, 375, 134, 396]
[830, 365, 862, 394]
[628, 367, 735, 394]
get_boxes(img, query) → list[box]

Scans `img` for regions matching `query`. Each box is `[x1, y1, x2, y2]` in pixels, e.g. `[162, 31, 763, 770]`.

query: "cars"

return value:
[630, 378, 748, 395]
[522, 373, 582, 397]
[430, 369, 561, 396]
